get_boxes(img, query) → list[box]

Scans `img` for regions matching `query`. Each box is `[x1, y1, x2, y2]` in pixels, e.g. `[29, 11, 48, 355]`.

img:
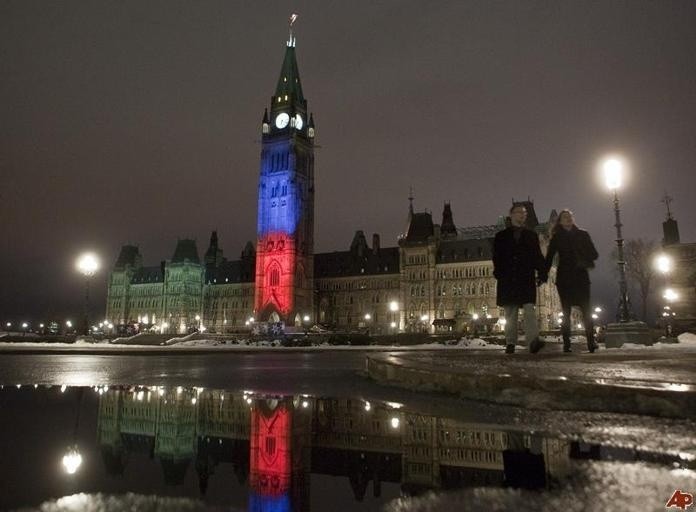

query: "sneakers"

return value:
[531, 337, 546, 354]
[505, 344, 515, 352]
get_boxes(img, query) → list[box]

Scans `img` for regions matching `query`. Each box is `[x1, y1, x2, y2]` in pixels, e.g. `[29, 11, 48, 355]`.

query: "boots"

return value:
[563, 332, 572, 351]
[586, 331, 598, 352]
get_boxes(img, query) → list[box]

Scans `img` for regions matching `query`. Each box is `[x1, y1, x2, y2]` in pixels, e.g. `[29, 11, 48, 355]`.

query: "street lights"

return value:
[604, 159, 630, 323]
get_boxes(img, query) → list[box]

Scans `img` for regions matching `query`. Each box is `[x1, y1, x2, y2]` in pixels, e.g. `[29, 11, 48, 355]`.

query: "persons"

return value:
[489, 202, 549, 354]
[535, 208, 600, 354]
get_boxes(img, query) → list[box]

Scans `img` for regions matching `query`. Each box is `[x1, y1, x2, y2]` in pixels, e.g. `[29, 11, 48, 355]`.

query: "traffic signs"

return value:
[76, 255, 99, 333]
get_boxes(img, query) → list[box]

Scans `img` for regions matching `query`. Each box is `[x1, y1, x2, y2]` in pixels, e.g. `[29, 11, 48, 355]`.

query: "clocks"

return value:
[273, 112, 304, 129]
